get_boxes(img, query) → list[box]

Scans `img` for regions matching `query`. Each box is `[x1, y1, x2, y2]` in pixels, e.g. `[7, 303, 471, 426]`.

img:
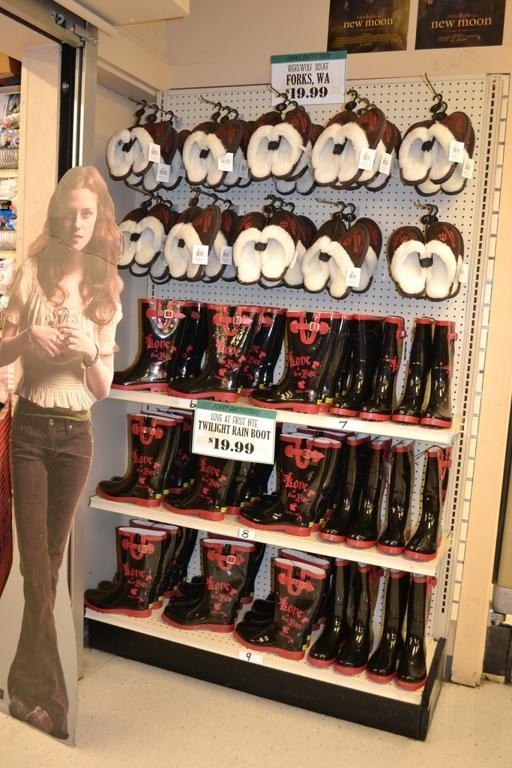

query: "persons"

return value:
[2, 164, 125, 747]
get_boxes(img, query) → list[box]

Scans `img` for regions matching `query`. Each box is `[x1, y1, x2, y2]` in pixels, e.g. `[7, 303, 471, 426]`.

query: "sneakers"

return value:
[24, 707, 56, 735]
[9, 696, 32, 722]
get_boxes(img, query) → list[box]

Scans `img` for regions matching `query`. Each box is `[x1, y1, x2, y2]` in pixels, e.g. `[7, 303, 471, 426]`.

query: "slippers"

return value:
[105, 108, 475, 195]
[116, 197, 464, 301]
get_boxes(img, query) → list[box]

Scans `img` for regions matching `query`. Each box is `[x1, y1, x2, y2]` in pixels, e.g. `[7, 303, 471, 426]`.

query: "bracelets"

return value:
[82, 342, 101, 368]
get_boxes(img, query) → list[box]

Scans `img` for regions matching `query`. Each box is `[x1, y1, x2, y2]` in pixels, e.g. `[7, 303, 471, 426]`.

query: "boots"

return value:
[94, 407, 453, 561]
[82, 292, 457, 428]
[84, 517, 438, 694]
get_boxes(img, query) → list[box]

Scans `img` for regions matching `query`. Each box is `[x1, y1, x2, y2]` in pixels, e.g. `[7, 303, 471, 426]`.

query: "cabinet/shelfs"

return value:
[82, 71, 510, 741]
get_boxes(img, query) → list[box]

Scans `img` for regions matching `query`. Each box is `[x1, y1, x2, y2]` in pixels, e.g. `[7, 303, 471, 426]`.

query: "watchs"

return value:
[26, 323, 35, 346]
